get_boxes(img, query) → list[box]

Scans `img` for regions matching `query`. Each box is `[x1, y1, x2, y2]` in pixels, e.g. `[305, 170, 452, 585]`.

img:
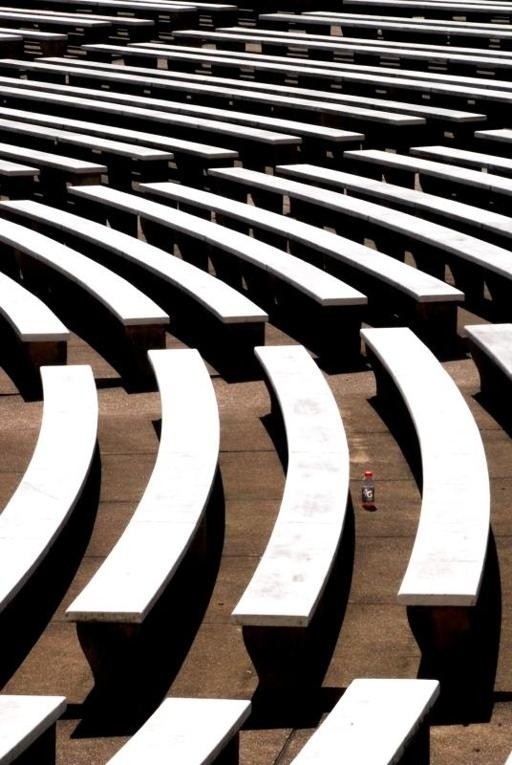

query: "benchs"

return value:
[0, 0, 512, 764]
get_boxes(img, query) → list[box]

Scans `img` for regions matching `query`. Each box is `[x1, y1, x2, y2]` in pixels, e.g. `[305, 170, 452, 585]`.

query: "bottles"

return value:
[360, 470, 378, 509]
